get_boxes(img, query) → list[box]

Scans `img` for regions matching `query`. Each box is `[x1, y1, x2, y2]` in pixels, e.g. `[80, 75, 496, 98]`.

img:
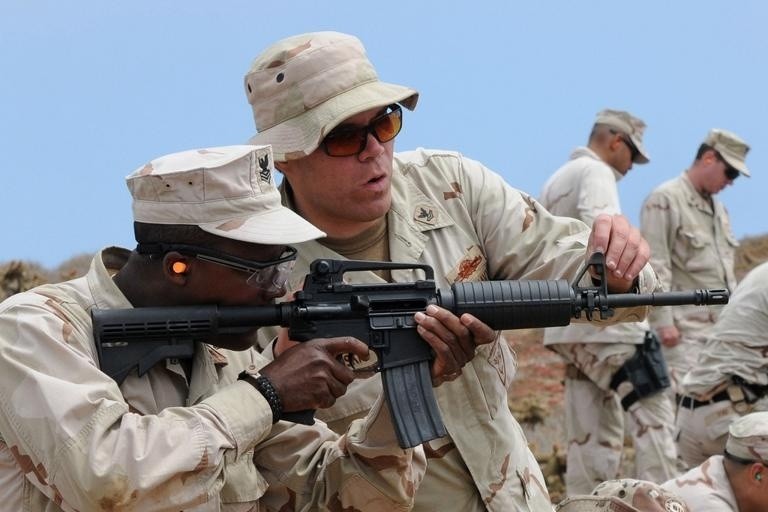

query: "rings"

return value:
[442, 373, 458, 377]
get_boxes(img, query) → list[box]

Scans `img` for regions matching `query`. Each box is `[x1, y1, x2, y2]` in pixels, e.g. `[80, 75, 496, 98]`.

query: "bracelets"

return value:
[237, 364, 282, 423]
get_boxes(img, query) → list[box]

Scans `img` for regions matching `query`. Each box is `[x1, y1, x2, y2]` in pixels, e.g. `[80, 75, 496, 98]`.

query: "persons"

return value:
[244, 29, 658, 512]
[660, 411, 768, 512]
[542, 107, 676, 497]
[0, 143, 498, 512]
[670, 262, 767, 469]
[639, 128, 751, 400]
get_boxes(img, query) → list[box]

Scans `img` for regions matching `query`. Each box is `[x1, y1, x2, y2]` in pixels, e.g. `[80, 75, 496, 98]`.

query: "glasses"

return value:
[709, 146, 739, 179]
[137, 242, 298, 292]
[318, 105, 403, 158]
[607, 129, 638, 163]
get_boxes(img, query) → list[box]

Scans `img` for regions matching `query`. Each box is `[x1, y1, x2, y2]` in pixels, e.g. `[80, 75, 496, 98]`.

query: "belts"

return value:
[676, 389, 730, 410]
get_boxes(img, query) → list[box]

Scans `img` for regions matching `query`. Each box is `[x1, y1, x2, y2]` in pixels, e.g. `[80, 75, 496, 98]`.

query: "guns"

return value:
[91, 253, 729, 450]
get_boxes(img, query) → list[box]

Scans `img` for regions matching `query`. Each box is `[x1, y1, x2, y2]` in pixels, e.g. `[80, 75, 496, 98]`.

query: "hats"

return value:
[593, 107, 652, 166]
[243, 31, 422, 164]
[726, 411, 768, 463]
[704, 129, 752, 179]
[127, 145, 327, 252]
